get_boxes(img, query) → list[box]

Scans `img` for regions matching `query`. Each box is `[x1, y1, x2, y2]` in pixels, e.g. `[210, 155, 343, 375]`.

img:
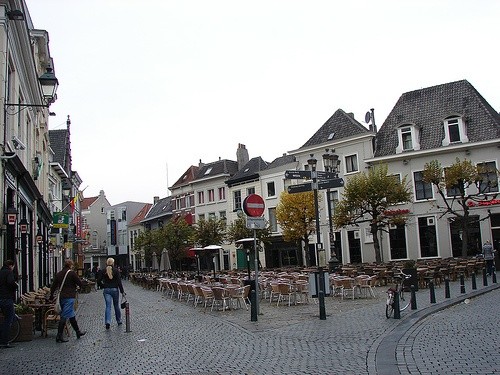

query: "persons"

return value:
[49, 259, 87, 343]
[97, 257, 127, 330]
[115, 264, 118, 268]
[117, 266, 123, 275]
[85, 265, 104, 290]
[483, 240, 496, 277]
[0, 260, 18, 348]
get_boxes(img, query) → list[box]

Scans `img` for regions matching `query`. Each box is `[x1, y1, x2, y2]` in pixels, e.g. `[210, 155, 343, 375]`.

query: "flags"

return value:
[71, 196, 80, 213]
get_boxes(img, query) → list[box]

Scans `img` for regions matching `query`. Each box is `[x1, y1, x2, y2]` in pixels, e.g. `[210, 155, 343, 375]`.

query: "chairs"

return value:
[128, 258, 494, 312]
[20, 286, 80, 336]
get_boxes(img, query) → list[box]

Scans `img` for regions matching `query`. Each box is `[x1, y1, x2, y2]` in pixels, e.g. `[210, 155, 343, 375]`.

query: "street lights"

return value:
[303, 153, 326, 320]
[321, 146, 343, 274]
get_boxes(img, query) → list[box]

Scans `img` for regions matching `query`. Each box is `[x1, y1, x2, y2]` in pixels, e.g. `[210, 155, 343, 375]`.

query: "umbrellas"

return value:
[188, 246, 204, 276]
[235, 237, 260, 279]
[159, 248, 172, 276]
[151, 251, 159, 272]
[203, 244, 222, 278]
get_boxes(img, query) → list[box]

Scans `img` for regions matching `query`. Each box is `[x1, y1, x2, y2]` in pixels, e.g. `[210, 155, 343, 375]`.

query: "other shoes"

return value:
[106, 324, 110, 330]
[117, 322, 122, 325]
[5, 343, 14, 348]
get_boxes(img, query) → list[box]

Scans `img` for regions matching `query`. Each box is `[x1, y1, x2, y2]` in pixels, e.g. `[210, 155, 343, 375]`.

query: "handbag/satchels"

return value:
[54, 295, 61, 313]
[121, 300, 127, 309]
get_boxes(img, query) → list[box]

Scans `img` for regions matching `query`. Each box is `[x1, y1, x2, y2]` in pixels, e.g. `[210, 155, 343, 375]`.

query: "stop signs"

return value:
[244, 193, 265, 217]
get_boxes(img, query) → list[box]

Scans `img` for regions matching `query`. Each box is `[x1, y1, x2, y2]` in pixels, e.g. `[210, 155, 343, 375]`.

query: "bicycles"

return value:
[385, 268, 412, 319]
[0, 304, 23, 348]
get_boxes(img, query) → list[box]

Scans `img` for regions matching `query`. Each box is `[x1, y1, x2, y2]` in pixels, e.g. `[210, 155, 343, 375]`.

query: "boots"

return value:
[55, 322, 69, 342]
[69, 316, 86, 339]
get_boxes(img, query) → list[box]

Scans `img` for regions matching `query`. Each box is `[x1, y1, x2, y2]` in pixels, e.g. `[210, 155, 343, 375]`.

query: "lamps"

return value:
[0, 135, 26, 159]
[4, 67, 58, 149]
